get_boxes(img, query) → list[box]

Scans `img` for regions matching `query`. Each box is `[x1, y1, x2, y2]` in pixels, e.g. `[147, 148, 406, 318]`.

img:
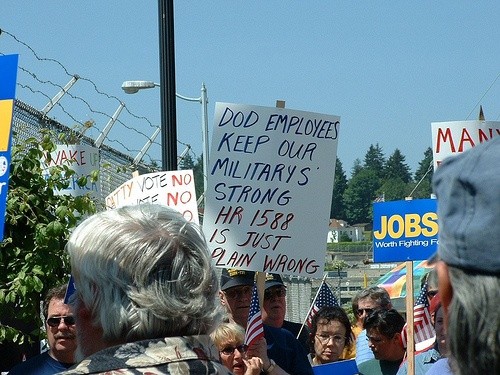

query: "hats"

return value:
[428, 136, 500, 272]
[220, 267, 257, 290]
[254, 271, 287, 290]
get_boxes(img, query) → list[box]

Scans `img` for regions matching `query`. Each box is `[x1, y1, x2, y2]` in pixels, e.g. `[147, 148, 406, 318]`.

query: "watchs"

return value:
[261, 359, 275, 373]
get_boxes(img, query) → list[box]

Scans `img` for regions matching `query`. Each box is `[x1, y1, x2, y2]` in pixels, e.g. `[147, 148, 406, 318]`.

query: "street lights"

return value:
[120, 81, 210, 206]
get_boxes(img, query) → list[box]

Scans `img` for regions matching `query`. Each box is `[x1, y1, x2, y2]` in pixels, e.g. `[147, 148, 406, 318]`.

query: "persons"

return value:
[7, 284, 81, 375]
[428, 271, 440, 304]
[335, 287, 392, 361]
[304, 307, 362, 375]
[262, 271, 311, 355]
[220, 266, 314, 375]
[395, 303, 449, 375]
[357, 308, 406, 375]
[210, 323, 290, 375]
[436, 135, 500, 375]
[52, 203, 235, 375]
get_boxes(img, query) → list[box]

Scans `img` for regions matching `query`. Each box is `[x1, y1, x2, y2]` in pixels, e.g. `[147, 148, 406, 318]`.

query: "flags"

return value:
[400, 281, 437, 355]
[305, 282, 339, 329]
[243, 281, 265, 347]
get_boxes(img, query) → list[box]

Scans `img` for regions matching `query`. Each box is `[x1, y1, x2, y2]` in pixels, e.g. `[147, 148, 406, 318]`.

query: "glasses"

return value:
[358, 308, 371, 314]
[264, 287, 286, 299]
[316, 332, 348, 344]
[219, 344, 248, 353]
[366, 337, 393, 345]
[45, 316, 75, 327]
